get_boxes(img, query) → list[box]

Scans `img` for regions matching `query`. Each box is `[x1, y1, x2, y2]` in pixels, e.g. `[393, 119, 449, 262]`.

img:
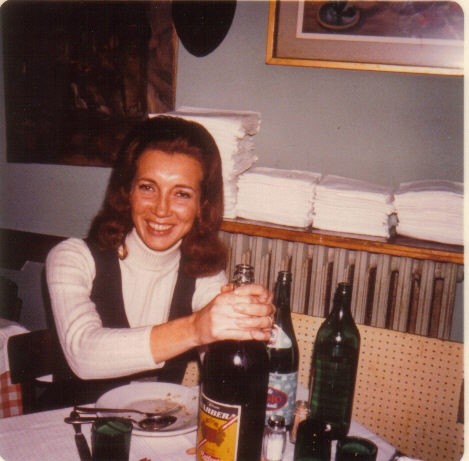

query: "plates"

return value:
[95, 382, 201, 437]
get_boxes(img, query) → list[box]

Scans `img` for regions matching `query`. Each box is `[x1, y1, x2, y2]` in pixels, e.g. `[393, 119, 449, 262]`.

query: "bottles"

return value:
[334, 436, 379, 461]
[292, 418, 333, 461]
[264, 270, 299, 435]
[195, 261, 268, 461]
[305, 282, 361, 439]
[262, 414, 287, 461]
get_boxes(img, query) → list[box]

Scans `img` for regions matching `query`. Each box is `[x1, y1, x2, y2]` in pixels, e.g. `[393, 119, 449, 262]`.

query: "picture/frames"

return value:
[265, 1, 464, 76]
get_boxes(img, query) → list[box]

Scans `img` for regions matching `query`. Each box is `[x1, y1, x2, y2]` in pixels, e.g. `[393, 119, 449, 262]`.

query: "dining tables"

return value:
[0, 383, 419, 461]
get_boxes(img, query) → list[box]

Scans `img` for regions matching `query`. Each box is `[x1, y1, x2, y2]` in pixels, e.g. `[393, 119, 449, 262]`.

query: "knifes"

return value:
[71, 411, 92, 461]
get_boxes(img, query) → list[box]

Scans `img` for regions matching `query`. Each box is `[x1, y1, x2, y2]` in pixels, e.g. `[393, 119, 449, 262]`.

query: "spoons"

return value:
[64, 414, 177, 431]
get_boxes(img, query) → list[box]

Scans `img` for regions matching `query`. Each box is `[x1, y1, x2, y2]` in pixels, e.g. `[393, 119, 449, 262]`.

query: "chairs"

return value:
[8, 328, 73, 414]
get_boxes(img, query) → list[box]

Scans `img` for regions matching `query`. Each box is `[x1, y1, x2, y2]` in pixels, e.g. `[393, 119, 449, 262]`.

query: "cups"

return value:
[91, 416, 133, 461]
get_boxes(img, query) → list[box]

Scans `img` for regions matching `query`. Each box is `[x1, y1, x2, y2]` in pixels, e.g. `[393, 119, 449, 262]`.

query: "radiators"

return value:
[218, 216, 464, 340]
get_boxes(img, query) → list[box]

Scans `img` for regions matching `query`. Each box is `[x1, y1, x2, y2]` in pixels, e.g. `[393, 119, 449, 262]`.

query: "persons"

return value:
[40, 115, 276, 410]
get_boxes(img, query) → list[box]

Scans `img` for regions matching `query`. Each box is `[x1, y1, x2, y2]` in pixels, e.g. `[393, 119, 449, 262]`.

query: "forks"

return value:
[73, 404, 182, 416]
